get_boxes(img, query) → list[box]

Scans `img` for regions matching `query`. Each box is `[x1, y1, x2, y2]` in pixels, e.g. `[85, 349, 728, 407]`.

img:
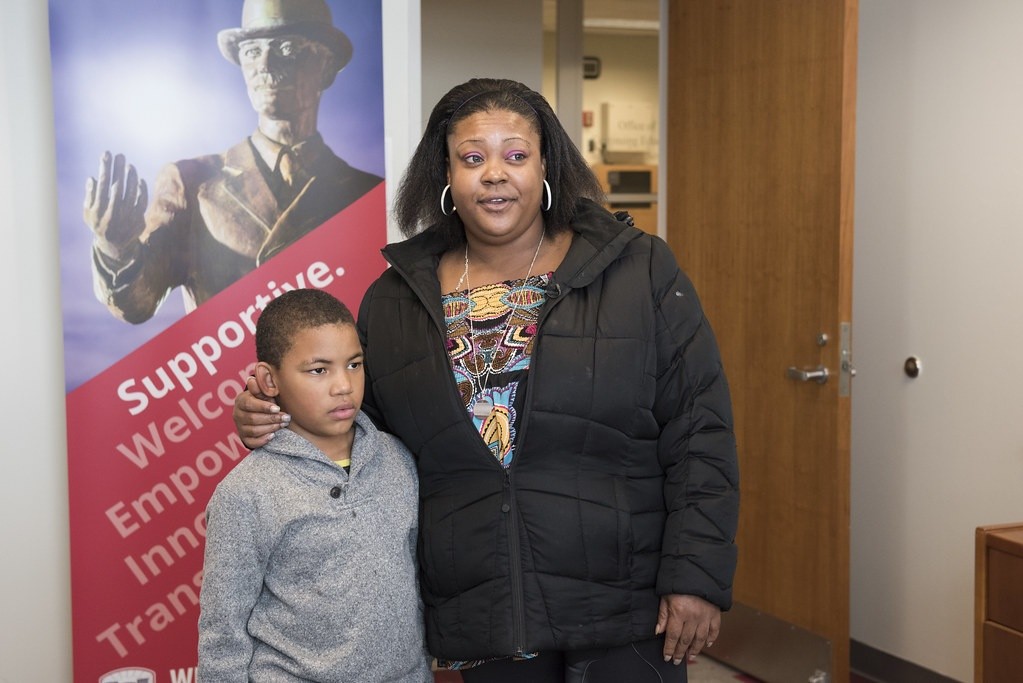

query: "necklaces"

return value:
[456, 223, 546, 421]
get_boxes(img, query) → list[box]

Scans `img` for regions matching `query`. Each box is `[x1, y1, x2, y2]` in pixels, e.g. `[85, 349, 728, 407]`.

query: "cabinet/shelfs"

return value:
[974, 522, 1023, 683]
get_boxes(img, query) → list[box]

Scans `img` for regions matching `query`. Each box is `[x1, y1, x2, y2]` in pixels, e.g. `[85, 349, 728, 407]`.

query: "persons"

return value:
[197, 289, 436, 683]
[83, 0, 385, 325]
[233, 79, 741, 683]
